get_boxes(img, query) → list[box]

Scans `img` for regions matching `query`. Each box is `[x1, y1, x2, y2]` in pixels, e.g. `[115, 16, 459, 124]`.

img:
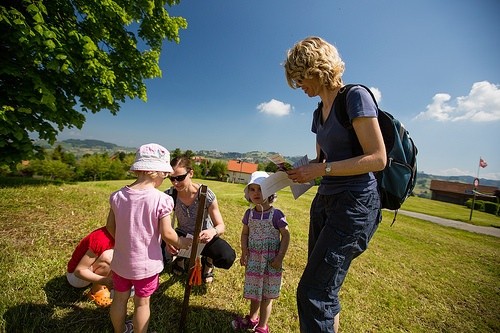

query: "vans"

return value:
[236, 178, 245, 184]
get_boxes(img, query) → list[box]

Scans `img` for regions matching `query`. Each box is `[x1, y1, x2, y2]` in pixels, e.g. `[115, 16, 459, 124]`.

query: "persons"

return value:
[162, 155, 236, 284]
[232, 171, 290, 333]
[66, 226, 117, 307]
[106, 143, 192, 333]
[284, 35, 387, 333]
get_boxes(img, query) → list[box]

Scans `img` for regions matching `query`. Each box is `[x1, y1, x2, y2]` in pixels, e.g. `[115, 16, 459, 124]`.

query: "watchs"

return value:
[325, 162, 331, 178]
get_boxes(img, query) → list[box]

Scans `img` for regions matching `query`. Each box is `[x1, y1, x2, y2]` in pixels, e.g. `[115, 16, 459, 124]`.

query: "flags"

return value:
[480, 158, 488, 168]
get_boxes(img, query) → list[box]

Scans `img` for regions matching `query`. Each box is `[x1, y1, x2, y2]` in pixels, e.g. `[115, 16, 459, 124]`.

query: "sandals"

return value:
[87, 285, 113, 308]
[201, 255, 214, 285]
[231, 315, 259, 330]
[253, 324, 269, 333]
[173, 257, 186, 277]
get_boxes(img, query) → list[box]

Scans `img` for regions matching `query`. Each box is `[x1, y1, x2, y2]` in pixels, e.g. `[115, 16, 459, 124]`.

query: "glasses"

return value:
[168, 169, 191, 181]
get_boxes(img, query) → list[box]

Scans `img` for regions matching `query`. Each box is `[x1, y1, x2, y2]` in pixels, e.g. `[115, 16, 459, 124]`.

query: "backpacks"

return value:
[317, 84, 417, 210]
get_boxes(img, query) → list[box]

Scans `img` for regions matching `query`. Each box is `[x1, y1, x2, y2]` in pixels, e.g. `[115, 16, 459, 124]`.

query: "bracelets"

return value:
[177, 236, 183, 248]
[213, 227, 219, 235]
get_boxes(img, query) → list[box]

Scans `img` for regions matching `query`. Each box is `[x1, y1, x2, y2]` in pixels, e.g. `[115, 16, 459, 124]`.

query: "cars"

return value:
[221, 177, 232, 182]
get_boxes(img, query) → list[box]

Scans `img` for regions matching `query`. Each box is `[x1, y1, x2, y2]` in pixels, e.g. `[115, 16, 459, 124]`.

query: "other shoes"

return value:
[122, 322, 134, 333]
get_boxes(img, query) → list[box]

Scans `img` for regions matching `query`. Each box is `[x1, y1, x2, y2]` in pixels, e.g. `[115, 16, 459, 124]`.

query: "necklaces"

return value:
[255, 206, 273, 212]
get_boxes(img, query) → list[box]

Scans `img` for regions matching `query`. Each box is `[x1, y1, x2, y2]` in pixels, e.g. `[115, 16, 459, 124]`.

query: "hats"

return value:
[244, 170, 277, 203]
[130, 142, 174, 173]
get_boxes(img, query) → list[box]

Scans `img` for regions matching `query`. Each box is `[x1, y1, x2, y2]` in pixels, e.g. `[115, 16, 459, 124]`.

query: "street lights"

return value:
[239, 153, 254, 183]
